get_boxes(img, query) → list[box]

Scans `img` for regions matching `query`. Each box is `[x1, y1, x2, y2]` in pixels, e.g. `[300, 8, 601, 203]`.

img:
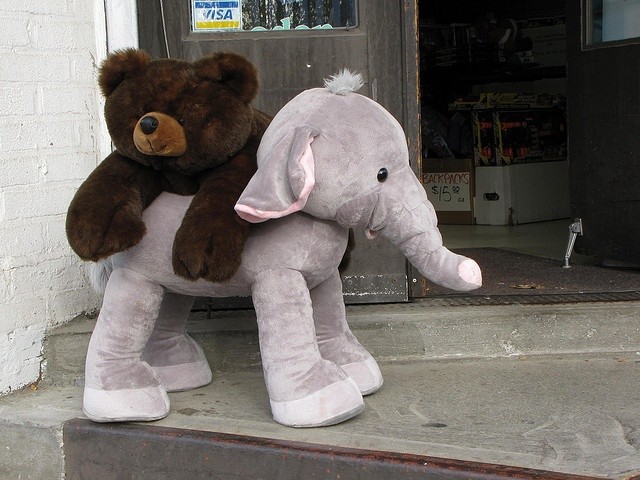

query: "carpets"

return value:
[427, 247, 640, 298]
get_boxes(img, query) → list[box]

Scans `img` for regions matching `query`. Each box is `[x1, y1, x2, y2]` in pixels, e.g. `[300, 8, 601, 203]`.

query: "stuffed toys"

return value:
[67, 48, 355, 283]
[82, 67, 482, 427]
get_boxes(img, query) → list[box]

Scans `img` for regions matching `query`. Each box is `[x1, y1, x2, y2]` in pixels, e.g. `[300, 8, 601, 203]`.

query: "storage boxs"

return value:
[422, 157, 474, 225]
[474, 159, 569, 225]
